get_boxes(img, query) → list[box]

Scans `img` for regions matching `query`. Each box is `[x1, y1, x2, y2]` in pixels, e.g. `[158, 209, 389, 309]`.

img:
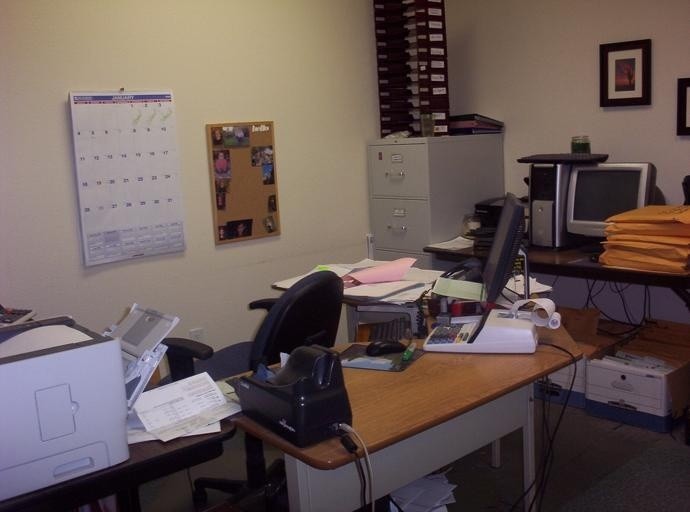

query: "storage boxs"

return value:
[536, 305, 688, 432]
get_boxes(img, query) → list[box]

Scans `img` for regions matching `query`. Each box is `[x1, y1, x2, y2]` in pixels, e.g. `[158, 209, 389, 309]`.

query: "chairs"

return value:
[149, 268, 346, 511]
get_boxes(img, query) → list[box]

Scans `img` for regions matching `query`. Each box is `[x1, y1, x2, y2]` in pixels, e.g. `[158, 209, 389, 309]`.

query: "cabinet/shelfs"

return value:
[364, 134, 505, 271]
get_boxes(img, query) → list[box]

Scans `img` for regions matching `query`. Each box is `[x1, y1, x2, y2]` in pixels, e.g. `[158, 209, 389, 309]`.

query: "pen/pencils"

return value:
[402, 342, 417, 361]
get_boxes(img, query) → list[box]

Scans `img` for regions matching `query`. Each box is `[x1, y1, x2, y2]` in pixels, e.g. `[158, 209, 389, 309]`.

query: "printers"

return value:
[1, 303, 178, 499]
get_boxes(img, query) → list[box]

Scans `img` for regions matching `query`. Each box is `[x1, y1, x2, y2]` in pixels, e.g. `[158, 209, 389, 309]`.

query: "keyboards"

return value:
[369, 316, 409, 340]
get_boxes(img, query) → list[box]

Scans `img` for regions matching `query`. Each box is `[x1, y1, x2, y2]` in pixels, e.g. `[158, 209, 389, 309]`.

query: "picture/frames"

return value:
[599, 37, 651, 110]
[675, 77, 690, 137]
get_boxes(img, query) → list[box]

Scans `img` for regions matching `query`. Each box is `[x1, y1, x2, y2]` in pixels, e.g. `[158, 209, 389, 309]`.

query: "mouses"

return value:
[366, 340, 407, 356]
[588, 252, 600, 263]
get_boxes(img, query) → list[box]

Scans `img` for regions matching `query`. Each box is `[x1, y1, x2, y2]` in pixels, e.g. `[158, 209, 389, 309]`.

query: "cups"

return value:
[421, 114, 435, 137]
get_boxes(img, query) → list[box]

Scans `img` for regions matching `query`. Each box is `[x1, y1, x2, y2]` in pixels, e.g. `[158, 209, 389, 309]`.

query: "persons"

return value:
[212, 127, 276, 241]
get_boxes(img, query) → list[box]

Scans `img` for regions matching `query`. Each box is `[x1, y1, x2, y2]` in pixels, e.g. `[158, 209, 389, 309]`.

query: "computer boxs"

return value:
[530, 163, 569, 248]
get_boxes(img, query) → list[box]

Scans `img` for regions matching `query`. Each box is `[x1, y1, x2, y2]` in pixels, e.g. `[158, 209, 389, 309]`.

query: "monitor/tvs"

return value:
[485, 190, 529, 304]
[565, 159, 669, 247]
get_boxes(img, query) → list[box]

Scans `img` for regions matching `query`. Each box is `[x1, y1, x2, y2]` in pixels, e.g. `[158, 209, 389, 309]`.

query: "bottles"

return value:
[571, 135, 591, 154]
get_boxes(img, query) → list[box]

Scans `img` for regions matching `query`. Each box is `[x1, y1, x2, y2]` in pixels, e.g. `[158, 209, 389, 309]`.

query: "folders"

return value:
[450, 113, 505, 133]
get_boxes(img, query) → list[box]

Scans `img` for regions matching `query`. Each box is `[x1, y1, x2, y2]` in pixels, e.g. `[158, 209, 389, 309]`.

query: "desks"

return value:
[1, 380, 237, 511]
[424, 232, 688, 311]
[211, 302, 584, 512]
